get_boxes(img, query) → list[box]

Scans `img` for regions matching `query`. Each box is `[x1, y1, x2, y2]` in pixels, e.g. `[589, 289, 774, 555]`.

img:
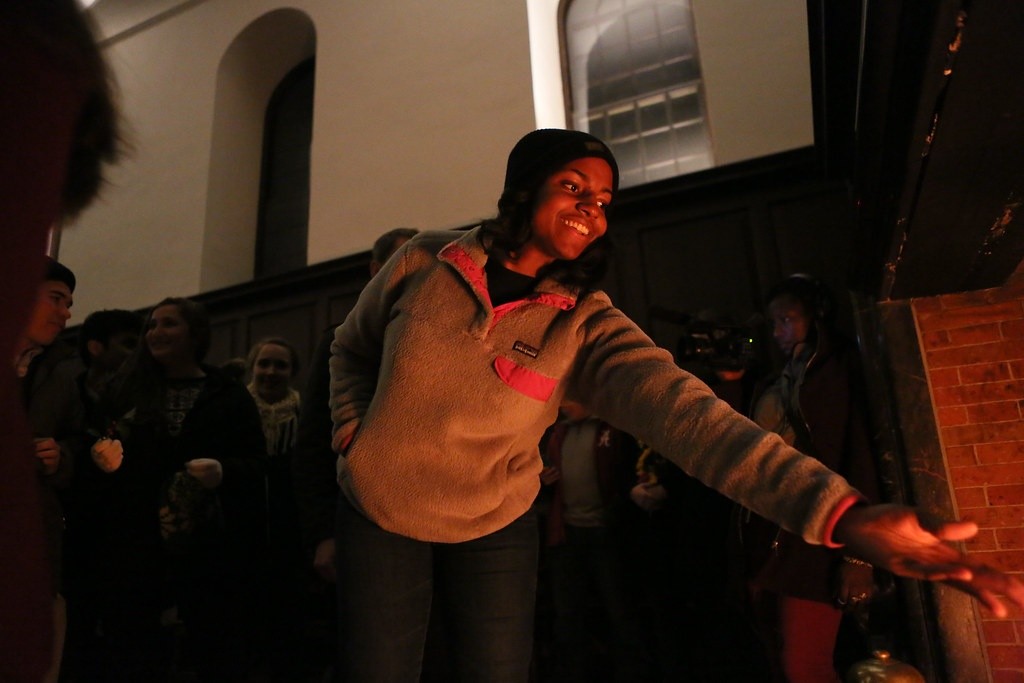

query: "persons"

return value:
[237, 335, 308, 683]
[284, 229, 426, 683]
[219, 356, 249, 386]
[60, 310, 151, 681]
[513, 318, 804, 682]
[328, 129, 1024, 683]
[83, 297, 248, 682]
[11, 255, 88, 682]
[744, 272, 882, 683]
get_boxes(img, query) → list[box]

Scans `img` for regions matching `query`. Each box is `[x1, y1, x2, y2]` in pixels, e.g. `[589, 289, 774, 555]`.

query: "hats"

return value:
[507, 128, 620, 196]
